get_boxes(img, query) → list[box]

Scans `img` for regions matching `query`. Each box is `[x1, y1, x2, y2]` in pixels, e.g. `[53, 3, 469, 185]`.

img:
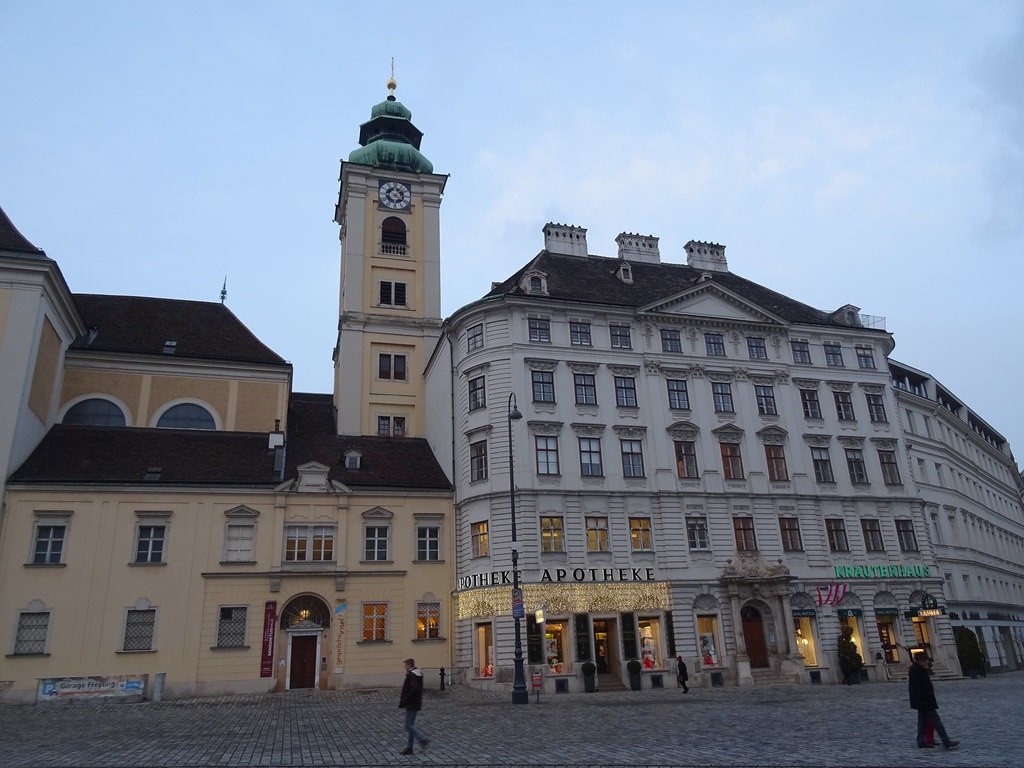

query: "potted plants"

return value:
[627, 661, 642, 690]
[956, 625, 982, 678]
[581, 662, 596, 693]
[837, 625, 862, 685]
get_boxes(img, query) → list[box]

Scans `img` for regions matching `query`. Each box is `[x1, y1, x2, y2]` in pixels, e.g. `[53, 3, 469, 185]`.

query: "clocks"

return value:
[378, 182, 411, 210]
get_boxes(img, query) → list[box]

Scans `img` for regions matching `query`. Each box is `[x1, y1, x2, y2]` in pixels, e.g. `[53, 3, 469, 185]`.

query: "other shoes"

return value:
[921, 743, 935, 748]
[683, 688, 689, 693]
[399, 747, 413, 755]
[420, 738, 430, 748]
[944, 740, 960, 748]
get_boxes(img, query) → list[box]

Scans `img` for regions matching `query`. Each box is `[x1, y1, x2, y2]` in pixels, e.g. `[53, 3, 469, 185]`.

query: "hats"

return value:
[914, 651, 929, 660]
[677, 655, 682, 661]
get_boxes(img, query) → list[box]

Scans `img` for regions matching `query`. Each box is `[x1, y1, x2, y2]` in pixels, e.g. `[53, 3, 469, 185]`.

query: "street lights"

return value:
[503, 388, 529, 707]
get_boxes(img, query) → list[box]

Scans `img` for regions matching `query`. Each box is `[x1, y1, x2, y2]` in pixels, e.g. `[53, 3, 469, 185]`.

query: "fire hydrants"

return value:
[439, 665, 446, 688]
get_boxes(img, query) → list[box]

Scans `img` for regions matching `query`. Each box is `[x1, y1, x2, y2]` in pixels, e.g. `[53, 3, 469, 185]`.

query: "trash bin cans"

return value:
[531, 673, 543, 691]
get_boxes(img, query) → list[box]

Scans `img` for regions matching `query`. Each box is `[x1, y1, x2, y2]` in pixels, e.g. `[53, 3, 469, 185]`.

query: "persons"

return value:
[676, 656, 689, 693]
[399, 658, 430, 755]
[976, 648, 987, 678]
[908, 653, 960, 749]
